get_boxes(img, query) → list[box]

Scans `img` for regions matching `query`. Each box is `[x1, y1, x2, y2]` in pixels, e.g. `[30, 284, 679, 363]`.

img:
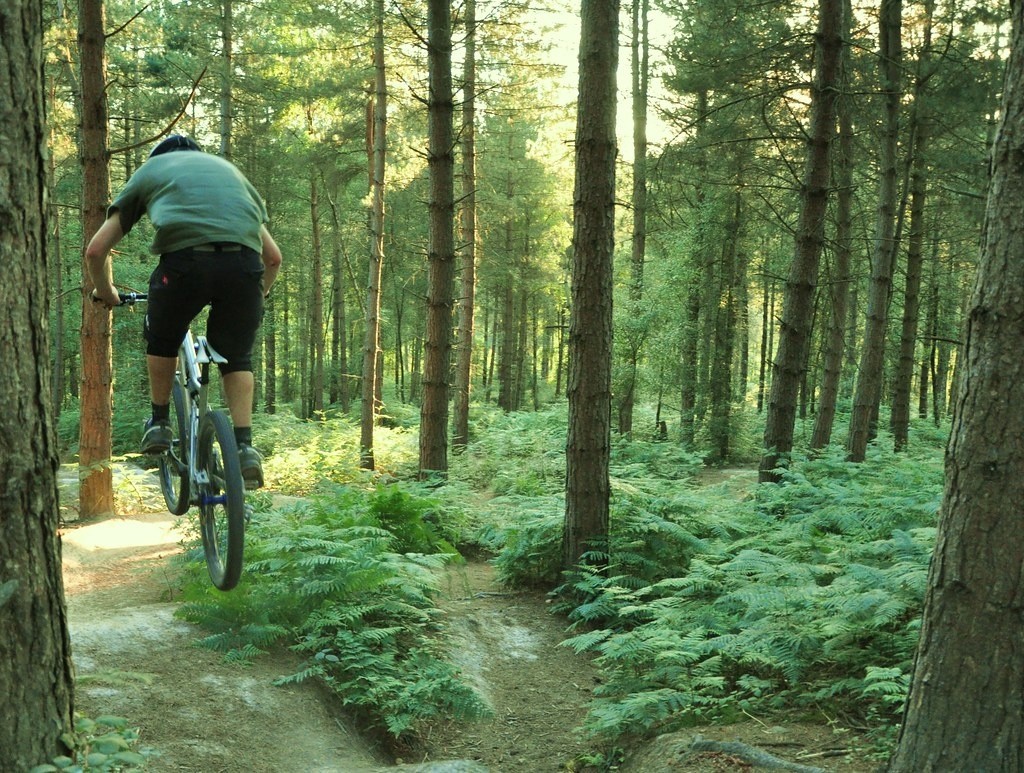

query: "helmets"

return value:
[149, 134, 202, 157]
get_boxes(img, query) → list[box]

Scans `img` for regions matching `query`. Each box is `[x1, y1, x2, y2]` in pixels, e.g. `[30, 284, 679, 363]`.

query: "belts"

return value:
[193, 244, 242, 251]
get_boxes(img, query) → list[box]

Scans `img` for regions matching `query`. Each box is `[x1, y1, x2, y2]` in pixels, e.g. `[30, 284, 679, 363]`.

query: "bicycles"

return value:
[91, 290, 271, 591]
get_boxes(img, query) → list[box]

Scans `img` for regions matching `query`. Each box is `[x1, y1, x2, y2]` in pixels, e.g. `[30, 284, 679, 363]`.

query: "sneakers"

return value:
[238, 443, 264, 490]
[140, 418, 174, 453]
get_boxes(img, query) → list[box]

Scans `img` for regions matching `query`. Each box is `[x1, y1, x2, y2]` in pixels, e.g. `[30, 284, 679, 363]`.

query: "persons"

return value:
[87, 134, 282, 488]
